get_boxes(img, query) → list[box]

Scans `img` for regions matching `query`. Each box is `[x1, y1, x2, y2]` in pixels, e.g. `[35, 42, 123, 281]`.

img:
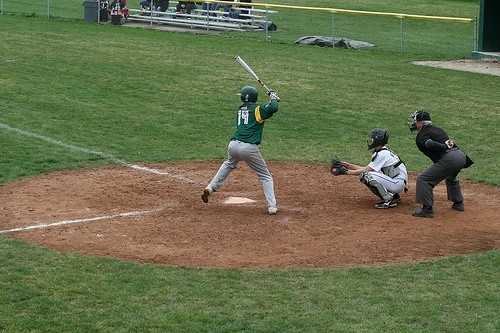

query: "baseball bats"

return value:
[234, 54, 281, 102]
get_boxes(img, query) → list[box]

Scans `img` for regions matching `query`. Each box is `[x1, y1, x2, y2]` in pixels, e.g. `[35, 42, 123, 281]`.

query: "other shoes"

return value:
[452, 201, 465, 211]
[412, 207, 434, 218]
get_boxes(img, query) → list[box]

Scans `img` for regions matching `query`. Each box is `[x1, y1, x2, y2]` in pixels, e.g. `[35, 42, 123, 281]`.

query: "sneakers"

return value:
[201, 187, 213, 204]
[378, 192, 401, 204]
[374, 200, 397, 208]
[268, 207, 278, 214]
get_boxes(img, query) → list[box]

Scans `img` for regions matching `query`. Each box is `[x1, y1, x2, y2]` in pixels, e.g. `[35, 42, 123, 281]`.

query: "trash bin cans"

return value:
[82, 0, 98, 22]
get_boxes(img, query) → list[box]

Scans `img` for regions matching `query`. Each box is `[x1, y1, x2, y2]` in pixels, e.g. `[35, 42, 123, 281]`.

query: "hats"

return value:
[414, 111, 431, 121]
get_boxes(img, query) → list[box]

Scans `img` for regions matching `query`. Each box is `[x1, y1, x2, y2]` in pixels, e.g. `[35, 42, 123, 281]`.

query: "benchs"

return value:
[127, 1, 278, 32]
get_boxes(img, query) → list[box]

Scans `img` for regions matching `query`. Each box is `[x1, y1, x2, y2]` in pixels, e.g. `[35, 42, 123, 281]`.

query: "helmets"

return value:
[237, 86, 258, 103]
[367, 129, 389, 150]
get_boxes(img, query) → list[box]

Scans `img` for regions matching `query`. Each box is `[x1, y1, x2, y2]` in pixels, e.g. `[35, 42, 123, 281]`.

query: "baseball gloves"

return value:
[331, 159, 348, 176]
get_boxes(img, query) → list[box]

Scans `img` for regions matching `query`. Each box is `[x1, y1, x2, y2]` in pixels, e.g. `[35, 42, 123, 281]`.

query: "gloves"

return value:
[269, 92, 278, 101]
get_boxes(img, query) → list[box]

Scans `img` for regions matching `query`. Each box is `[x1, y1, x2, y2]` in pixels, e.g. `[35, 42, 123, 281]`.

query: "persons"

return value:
[107, 0, 131, 21]
[408, 108, 467, 219]
[201, 85, 279, 214]
[330, 127, 409, 210]
[138, 0, 253, 24]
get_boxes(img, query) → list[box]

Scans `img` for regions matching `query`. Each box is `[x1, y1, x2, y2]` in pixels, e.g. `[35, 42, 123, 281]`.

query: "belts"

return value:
[404, 180, 407, 186]
[231, 139, 246, 143]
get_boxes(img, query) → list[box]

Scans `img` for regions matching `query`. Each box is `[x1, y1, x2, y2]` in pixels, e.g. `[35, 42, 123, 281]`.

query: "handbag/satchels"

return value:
[461, 156, 473, 169]
